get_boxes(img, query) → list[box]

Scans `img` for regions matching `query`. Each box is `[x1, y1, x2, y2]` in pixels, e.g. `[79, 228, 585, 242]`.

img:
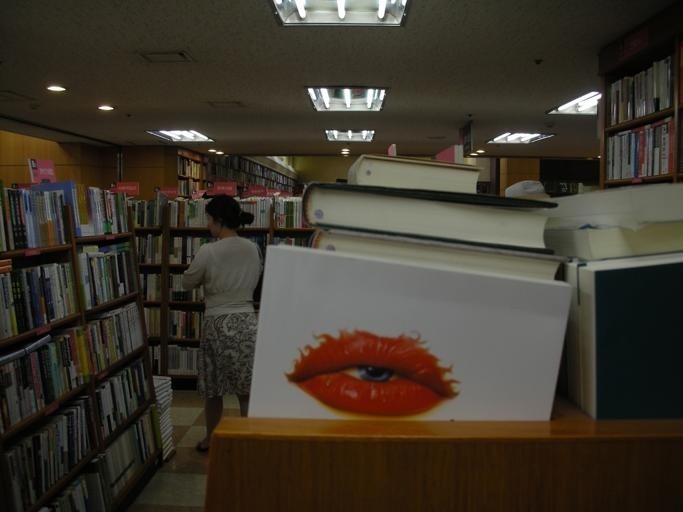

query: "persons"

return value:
[182, 193, 264, 456]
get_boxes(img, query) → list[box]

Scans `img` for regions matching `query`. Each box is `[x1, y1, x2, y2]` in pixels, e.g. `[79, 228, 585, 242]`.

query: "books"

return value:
[178, 151, 296, 187]
[191, 285, 203, 302]
[300, 181, 559, 255]
[543, 221, 683, 262]
[246, 241, 573, 420]
[149, 344, 161, 377]
[127, 195, 163, 228]
[170, 273, 181, 303]
[38, 406, 154, 512]
[537, 183, 683, 232]
[249, 235, 264, 259]
[168, 197, 182, 230]
[577, 251, 682, 419]
[265, 196, 300, 229]
[607, 117, 671, 182]
[166, 345, 182, 376]
[152, 374, 175, 462]
[564, 256, 587, 411]
[31, 180, 128, 237]
[182, 347, 200, 376]
[0, 356, 151, 512]
[0, 259, 77, 343]
[186, 236, 220, 264]
[168, 308, 182, 339]
[0, 179, 70, 252]
[607, 56, 671, 127]
[143, 306, 160, 337]
[177, 180, 248, 197]
[346, 152, 484, 195]
[1, 301, 146, 436]
[182, 311, 203, 340]
[169, 236, 181, 266]
[140, 274, 161, 302]
[182, 197, 265, 228]
[267, 233, 300, 247]
[177, 156, 294, 194]
[135, 233, 162, 265]
[77, 241, 136, 310]
[306, 229, 568, 281]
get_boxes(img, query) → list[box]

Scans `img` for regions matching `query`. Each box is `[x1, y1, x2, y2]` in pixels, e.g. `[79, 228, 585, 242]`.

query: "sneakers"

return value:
[196, 442, 210, 451]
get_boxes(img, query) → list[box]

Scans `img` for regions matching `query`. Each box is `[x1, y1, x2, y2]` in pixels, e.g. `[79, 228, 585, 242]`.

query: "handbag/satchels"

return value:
[252, 243, 263, 310]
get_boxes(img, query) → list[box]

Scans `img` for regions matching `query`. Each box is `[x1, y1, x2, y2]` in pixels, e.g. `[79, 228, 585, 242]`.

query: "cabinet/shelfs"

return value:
[123, 144, 301, 201]
[129, 202, 306, 391]
[1, 205, 161, 509]
[599, 44, 682, 189]
